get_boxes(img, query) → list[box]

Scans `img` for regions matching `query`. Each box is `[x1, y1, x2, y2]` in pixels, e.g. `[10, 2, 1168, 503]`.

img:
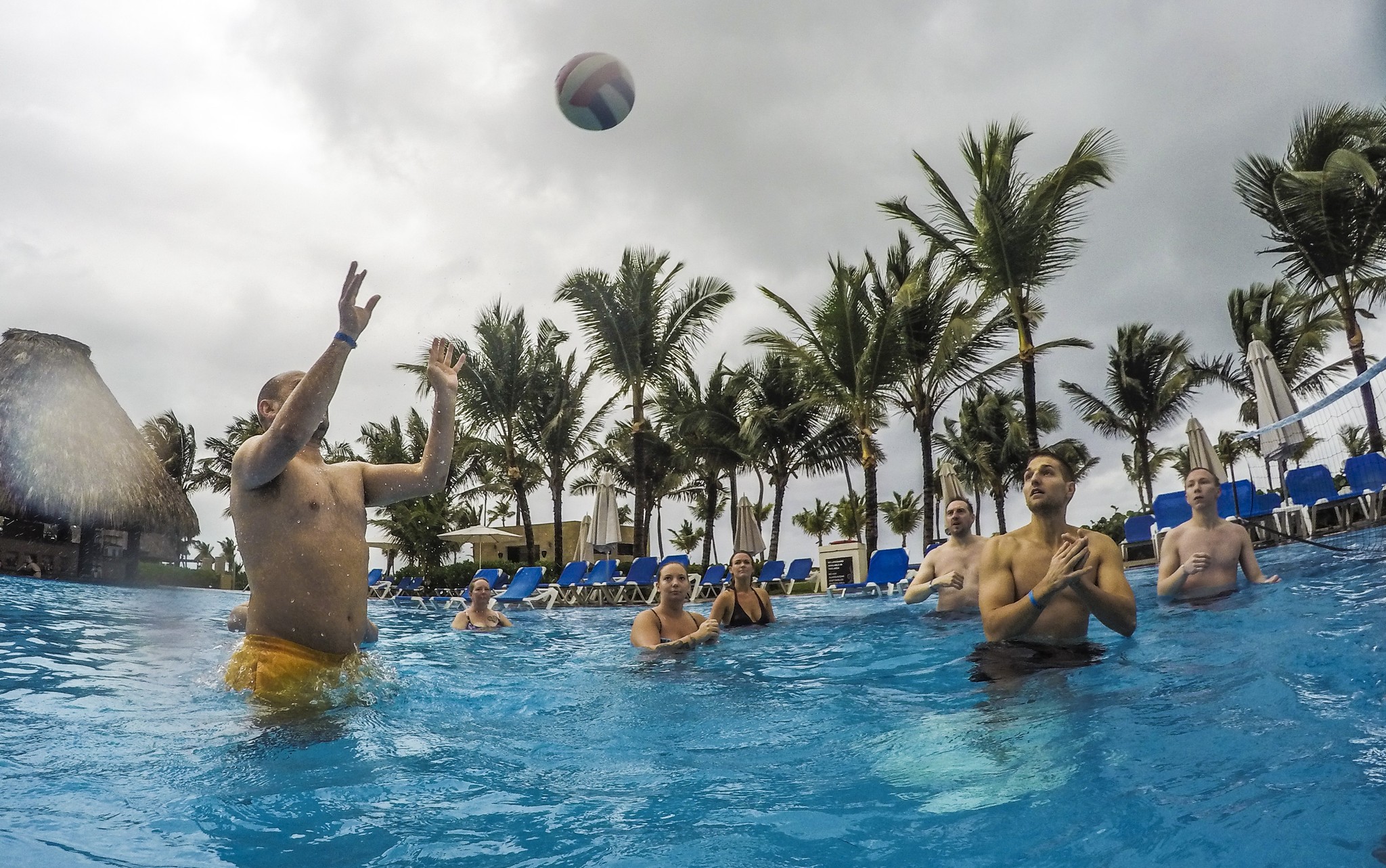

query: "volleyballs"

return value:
[554, 51, 635, 130]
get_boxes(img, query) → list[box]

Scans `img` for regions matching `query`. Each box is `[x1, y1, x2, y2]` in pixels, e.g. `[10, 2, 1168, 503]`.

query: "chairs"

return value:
[368, 554, 822, 611]
[826, 543, 943, 598]
[1119, 451, 1386, 561]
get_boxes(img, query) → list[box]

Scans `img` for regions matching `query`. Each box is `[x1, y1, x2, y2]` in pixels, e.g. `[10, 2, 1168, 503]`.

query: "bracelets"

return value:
[929, 580, 938, 591]
[1029, 590, 1045, 610]
[334, 332, 356, 350]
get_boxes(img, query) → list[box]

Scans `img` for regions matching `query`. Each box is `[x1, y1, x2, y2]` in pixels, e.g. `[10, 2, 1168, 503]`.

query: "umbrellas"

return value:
[586, 471, 623, 559]
[573, 512, 595, 573]
[1185, 412, 1229, 484]
[366, 536, 410, 576]
[939, 463, 965, 504]
[1245, 332, 1306, 506]
[732, 493, 766, 558]
[436, 525, 523, 569]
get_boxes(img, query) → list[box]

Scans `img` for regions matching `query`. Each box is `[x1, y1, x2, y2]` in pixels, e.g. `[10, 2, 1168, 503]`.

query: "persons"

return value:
[452, 577, 513, 630]
[979, 449, 1137, 642]
[1157, 468, 1281, 599]
[904, 497, 990, 611]
[630, 561, 720, 650]
[16, 553, 41, 577]
[225, 260, 465, 695]
[709, 551, 775, 626]
